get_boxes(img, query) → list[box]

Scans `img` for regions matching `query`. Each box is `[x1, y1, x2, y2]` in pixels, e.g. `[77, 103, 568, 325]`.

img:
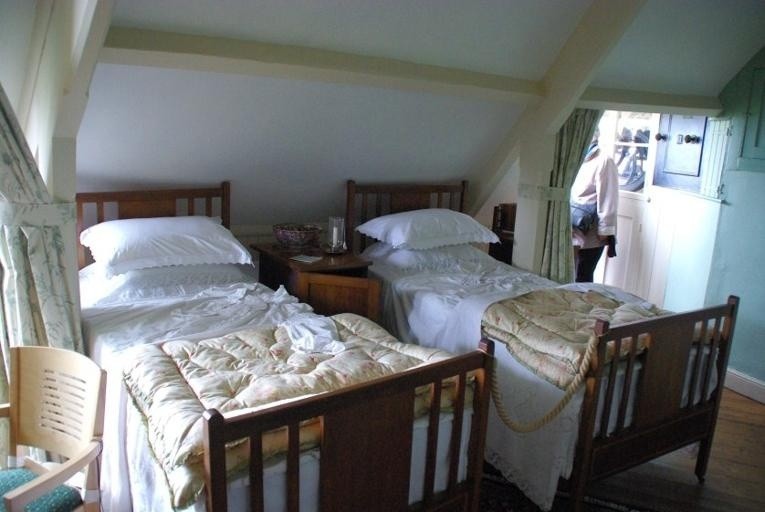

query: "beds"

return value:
[347, 179, 740, 510]
[76, 181, 495, 512]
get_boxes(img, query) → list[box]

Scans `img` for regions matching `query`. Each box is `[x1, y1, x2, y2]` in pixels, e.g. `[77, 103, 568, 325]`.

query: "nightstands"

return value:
[249, 242, 382, 322]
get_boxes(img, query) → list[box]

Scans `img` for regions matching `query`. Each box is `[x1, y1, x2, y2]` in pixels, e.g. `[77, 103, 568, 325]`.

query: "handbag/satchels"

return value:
[569, 200, 597, 233]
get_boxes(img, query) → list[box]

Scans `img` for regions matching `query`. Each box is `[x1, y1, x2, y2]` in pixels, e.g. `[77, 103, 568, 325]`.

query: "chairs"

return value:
[0, 346, 107, 512]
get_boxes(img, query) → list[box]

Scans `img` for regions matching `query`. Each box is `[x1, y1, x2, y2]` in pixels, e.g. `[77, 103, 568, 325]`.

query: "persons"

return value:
[568, 125, 617, 281]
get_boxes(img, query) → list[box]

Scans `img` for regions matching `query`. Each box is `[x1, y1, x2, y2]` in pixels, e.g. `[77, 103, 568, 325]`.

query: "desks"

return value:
[488, 202, 517, 265]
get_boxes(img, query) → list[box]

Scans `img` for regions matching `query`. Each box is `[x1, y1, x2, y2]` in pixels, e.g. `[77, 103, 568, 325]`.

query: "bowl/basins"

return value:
[273, 224, 323, 245]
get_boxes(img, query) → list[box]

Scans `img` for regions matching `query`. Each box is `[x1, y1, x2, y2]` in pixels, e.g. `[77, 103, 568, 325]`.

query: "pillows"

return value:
[79, 261, 251, 307]
[79, 216, 256, 278]
[354, 208, 503, 250]
[361, 241, 496, 276]
